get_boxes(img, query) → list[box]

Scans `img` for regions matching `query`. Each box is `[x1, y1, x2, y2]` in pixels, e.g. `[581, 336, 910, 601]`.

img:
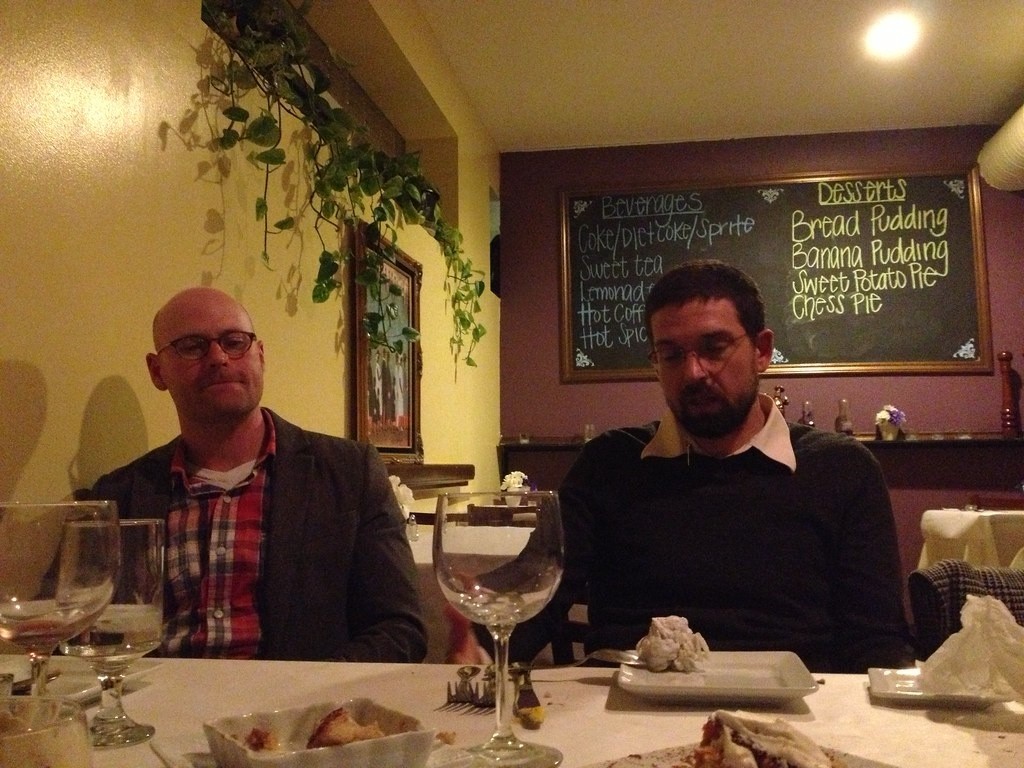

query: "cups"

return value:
[519, 433, 529, 444]
[504, 495, 521, 506]
[1, 696, 93, 768]
[583, 424, 594, 442]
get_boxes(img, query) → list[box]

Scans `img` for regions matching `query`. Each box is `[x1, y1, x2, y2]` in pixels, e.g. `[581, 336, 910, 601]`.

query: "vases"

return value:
[880, 425, 899, 441]
[507, 491, 522, 505]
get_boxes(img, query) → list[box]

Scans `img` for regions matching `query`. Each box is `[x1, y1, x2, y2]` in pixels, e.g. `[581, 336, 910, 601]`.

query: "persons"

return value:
[17, 287, 429, 664]
[444, 260, 908, 676]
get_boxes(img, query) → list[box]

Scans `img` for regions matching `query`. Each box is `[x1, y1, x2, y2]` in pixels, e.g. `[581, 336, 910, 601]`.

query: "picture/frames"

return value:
[346, 217, 424, 463]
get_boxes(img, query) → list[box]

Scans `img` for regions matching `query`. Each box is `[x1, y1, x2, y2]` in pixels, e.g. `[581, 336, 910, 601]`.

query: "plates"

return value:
[1, 655, 166, 712]
[866, 666, 1017, 706]
[201, 696, 437, 768]
[618, 649, 820, 705]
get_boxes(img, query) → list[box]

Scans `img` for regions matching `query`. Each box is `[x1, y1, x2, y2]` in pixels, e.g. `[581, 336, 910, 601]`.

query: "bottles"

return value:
[834, 399, 853, 438]
[797, 401, 817, 428]
[409, 514, 419, 542]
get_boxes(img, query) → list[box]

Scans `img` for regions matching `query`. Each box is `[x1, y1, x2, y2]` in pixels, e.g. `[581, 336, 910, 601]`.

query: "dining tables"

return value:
[402, 492, 568, 587]
[0, 655, 1024, 768]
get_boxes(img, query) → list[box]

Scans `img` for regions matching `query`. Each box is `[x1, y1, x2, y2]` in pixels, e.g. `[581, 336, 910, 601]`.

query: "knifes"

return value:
[517, 662, 544, 724]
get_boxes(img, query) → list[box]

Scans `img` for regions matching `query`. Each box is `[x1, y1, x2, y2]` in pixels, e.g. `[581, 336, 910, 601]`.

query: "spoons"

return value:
[509, 647, 647, 670]
[510, 662, 524, 717]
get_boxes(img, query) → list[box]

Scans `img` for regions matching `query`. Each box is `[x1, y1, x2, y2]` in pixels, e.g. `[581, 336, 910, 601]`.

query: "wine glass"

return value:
[433, 490, 566, 768]
[0, 500, 121, 729]
[57, 520, 167, 751]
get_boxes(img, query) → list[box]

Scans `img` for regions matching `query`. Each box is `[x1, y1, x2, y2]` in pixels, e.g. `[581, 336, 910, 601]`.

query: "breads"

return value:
[308, 707, 385, 748]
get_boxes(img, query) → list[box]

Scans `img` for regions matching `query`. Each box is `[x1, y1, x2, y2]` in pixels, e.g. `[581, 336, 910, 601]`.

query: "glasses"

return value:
[155, 330, 257, 361]
[647, 330, 749, 372]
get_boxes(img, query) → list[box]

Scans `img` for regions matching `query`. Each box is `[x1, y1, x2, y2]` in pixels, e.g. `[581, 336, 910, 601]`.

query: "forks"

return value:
[447, 665, 481, 702]
[464, 664, 496, 708]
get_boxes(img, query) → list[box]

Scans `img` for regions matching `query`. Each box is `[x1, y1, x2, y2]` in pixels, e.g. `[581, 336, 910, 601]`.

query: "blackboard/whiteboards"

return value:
[558, 162, 997, 385]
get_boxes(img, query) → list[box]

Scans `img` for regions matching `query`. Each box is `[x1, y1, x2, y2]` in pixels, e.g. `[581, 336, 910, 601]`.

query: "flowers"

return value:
[391, 475, 411, 519]
[875, 407, 903, 424]
[500, 473, 531, 485]
